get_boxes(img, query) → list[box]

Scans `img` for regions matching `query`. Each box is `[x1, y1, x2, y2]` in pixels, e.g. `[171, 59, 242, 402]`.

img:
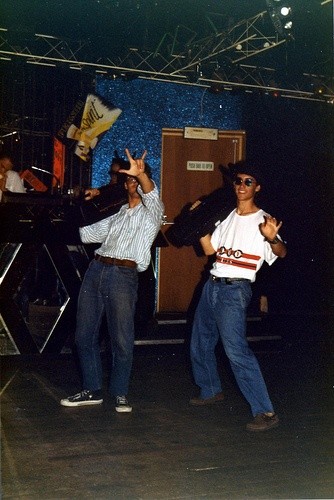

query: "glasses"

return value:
[234, 177, 256, 186]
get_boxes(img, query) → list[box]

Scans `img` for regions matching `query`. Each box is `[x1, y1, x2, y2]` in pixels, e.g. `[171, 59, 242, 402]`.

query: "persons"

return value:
[0, 155, 25, 200]
[189, 160, 287, 431]
[61, 148, 164, 412]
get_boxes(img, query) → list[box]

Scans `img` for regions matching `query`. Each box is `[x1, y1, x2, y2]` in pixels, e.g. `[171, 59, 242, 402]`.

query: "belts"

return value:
[212, 275, 249, 282]
[96, 254, 137, 267]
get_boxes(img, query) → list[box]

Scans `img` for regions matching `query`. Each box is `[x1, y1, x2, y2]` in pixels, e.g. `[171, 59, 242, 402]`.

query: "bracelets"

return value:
[269, 238, 278, 244]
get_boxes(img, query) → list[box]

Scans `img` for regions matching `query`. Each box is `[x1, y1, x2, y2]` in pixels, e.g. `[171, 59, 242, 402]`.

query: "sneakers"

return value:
[189, 393, 225, 406]
[245, 413, 279, 431]
[60, 388, 103, 406]
[115, 395, 132, 412]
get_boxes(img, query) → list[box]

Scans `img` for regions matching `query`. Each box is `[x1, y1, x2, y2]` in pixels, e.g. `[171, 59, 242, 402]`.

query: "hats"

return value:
[227, 161, 260, 184]
